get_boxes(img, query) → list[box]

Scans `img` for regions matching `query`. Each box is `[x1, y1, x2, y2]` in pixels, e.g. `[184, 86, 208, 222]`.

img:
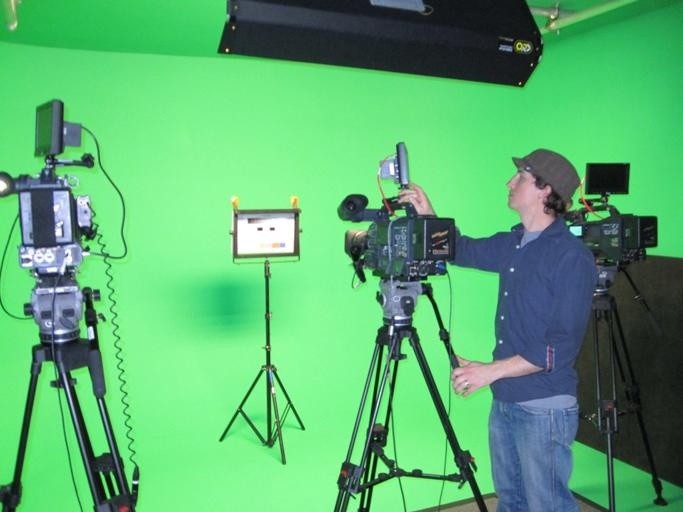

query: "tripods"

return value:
[573, 258, 669, 511]
[220, 261, 306, 464]
[334, 279, 490, 512]
[0, 287, 136, 512]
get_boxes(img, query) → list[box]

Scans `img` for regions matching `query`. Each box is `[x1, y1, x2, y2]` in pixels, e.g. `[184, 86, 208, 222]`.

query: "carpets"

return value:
[414, 489, 609, 512]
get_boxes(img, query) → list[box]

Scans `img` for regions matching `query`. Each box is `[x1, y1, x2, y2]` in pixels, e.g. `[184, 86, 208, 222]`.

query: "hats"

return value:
[512, 149, 581, 205]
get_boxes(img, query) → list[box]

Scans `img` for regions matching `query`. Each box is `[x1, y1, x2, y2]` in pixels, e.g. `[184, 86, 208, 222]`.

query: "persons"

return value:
[394, 147, 599, 512]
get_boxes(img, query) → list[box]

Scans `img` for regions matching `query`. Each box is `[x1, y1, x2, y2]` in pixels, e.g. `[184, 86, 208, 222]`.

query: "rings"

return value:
[464, 379, 470, 387]
